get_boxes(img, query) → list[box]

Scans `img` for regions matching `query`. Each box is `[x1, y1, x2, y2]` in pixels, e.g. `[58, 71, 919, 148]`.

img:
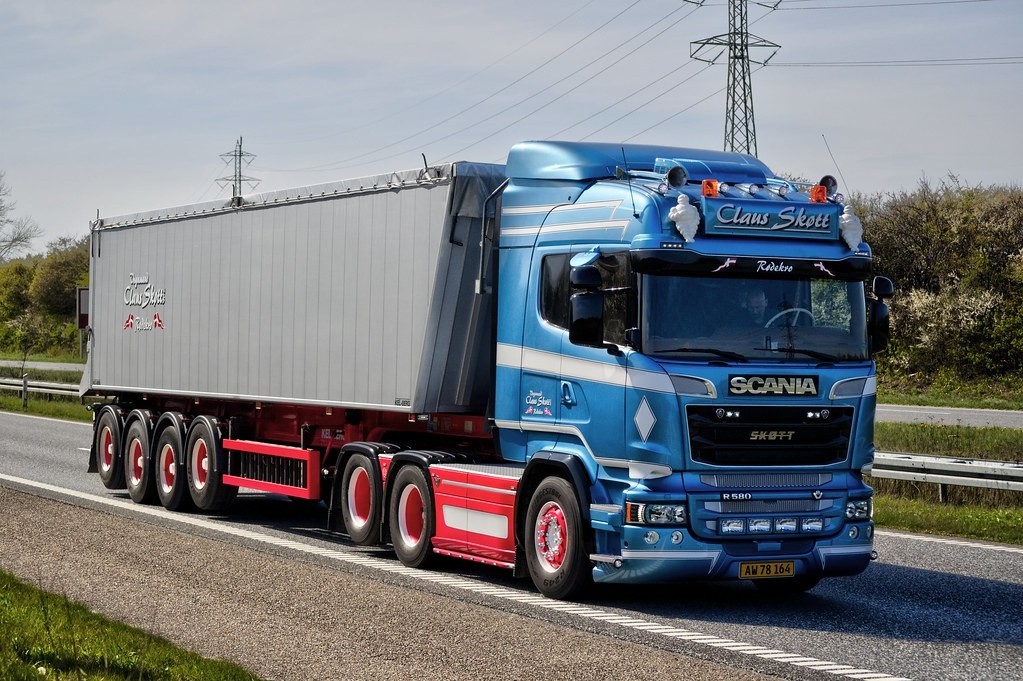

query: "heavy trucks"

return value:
[78, 139, 896, 602]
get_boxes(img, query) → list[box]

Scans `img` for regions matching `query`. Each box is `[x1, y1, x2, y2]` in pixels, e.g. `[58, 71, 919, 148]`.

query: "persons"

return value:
[720, 288, 797, 343]
